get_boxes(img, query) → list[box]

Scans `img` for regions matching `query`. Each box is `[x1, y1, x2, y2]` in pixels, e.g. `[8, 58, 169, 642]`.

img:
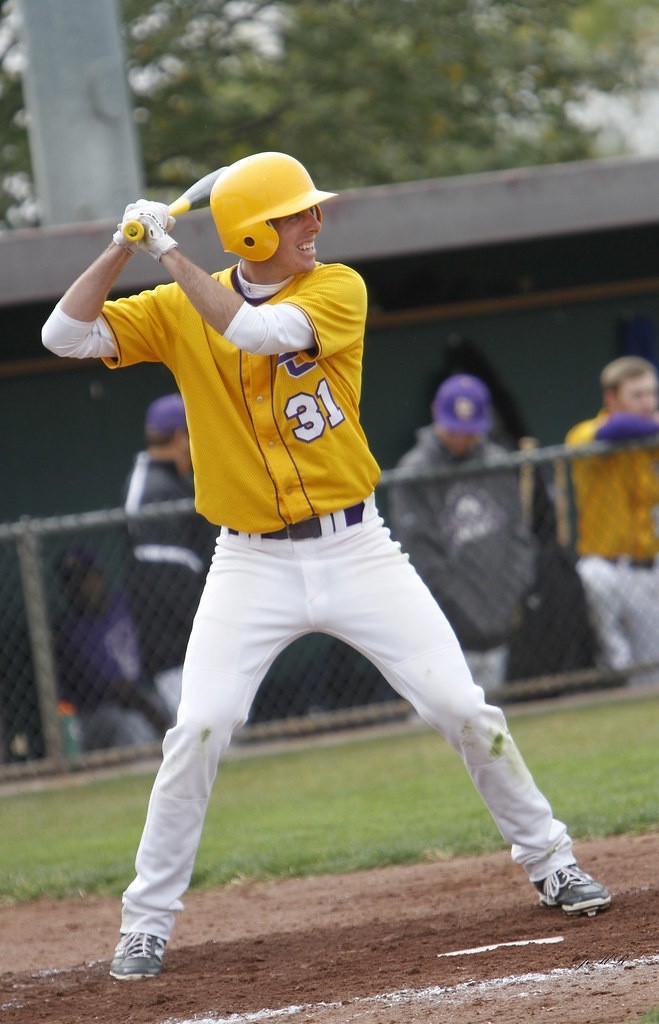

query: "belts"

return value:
[605, 556, 651, 570]
[227, 499, 364, 540]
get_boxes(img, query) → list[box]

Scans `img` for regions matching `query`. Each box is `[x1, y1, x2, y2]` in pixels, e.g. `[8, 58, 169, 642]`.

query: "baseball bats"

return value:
[123, 164, 230, 242]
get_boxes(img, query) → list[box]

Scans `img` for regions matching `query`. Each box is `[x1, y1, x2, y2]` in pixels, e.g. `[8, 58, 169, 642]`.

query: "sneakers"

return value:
[532, 862, 613, 916]
[108, 931, 165, 980]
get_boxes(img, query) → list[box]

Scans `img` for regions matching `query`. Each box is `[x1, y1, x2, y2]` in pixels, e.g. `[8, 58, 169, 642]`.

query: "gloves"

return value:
[123, 205, 179, 261]
[113, 197, 177, 256]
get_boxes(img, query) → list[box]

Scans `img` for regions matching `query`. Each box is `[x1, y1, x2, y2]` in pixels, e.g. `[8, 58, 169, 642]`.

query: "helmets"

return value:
[209, 152, 338, 261]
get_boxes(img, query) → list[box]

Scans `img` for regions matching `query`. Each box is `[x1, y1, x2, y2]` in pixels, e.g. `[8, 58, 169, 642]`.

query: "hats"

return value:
[141, 393, 186, 430]
[431, 374, 498, 434]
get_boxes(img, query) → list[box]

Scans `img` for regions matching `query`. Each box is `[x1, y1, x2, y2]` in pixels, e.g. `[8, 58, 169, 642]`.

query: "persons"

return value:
[120, 396, 205, 728]
[37, 152, 613, 981]
[49, 543, 169, 741]
[384, 375, 534, 687]
[558, 352, 659, 666]
